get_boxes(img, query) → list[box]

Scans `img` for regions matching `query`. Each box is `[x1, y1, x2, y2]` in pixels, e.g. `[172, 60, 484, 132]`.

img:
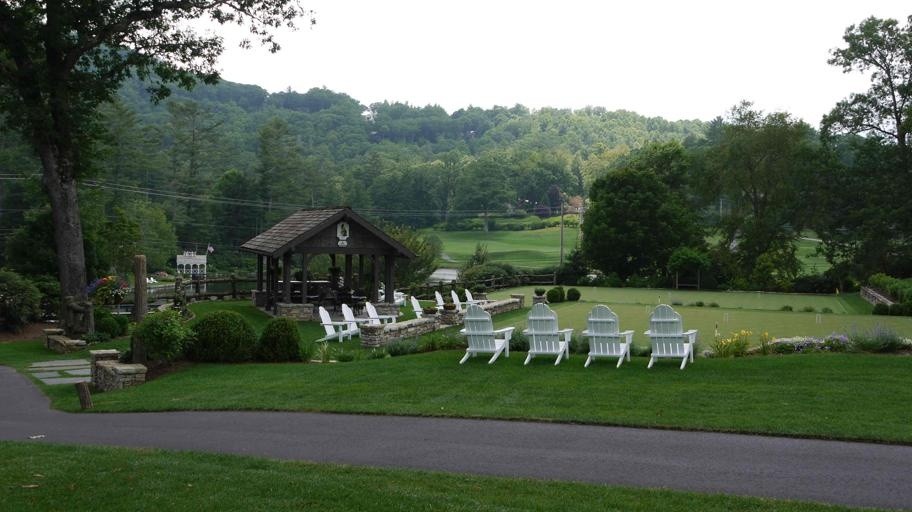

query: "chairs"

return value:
[457, 303, 697, 371]
[317, 288, 495, 345]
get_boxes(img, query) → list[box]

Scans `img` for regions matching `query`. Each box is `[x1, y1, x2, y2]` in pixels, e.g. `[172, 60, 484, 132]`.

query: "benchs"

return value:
[40, 327, 85, 355]
[88, 348, 147, 391]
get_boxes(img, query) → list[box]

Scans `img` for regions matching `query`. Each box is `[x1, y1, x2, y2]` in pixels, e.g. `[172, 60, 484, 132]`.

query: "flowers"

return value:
[85, 275, 131, 305]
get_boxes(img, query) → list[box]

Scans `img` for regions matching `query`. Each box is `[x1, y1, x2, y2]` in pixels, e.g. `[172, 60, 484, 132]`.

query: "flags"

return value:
[207, 243, 215, 254]
